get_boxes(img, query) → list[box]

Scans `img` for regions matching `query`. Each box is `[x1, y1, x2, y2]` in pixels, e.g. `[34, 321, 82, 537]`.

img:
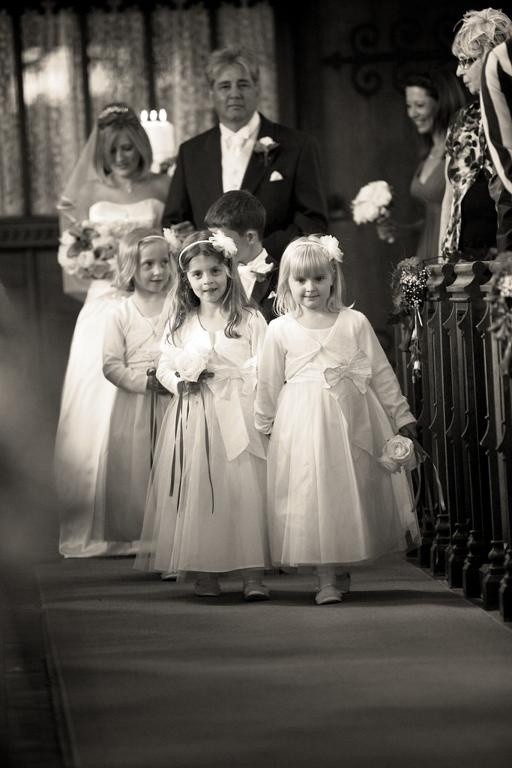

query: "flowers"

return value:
[58, 221, 117, 281]
[176, 352, 214, 387]
[402, 269, 432, 313]
[350, 179, 396, 245]
[378, 435, 426, 478]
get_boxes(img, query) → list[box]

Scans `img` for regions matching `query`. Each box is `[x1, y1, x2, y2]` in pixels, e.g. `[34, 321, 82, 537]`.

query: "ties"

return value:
[225, 133, 245, 182]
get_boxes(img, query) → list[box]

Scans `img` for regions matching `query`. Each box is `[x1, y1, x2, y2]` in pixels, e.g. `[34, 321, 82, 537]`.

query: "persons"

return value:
[131, 230, 268, 600]
[376, 8, 512, 266]
[252, 233, 420, 605]
[54, 100, 196, 559]
[203, 190, 280, 324]
[161, 44, 329, 259]
[91, 227, 174, 582]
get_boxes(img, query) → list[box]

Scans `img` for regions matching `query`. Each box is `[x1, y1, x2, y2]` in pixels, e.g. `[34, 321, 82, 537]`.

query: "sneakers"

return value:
[314, 572, 350, 605]
[242, 579, 270, 602]
[192, 575, 222, 597]
[159, 572, 184, 582]
